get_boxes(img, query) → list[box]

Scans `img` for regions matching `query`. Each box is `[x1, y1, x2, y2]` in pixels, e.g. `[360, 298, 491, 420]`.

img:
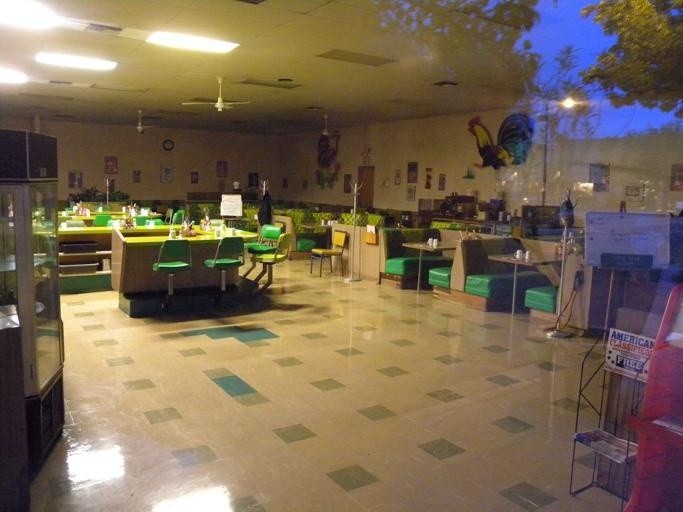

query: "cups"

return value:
[517, 250, 530, 262]
[149, 220, 155, 228]
[429, 238, 438, 248]
[321, 218, 338, 226]
[215, 223, 236, 237]
[60, 205, 148, 217]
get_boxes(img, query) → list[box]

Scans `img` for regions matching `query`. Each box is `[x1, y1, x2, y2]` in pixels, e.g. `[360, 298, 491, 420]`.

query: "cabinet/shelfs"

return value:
[1, 130, 65, 466]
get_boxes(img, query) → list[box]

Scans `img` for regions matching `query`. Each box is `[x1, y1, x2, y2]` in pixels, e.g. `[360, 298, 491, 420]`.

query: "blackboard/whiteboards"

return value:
[220, 194, 243, 216]
[585, 212, 670, 269]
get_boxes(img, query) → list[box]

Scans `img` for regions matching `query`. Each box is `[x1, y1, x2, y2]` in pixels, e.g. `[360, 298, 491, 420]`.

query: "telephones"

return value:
[573, 271, 585, 291]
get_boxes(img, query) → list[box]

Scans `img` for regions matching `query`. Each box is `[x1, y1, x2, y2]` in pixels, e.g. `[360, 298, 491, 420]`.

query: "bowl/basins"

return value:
[83, 219, 94, 227]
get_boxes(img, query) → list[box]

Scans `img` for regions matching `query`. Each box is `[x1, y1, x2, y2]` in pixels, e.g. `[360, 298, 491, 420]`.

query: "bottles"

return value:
[169, 228, 177, 239]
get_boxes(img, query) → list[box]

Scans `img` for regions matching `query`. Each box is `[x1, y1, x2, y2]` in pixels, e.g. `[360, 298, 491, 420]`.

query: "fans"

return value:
[181, 75, 251, 112]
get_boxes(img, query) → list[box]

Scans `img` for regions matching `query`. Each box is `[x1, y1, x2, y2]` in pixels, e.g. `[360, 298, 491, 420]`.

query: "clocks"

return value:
[162, 139, 174, 151]
